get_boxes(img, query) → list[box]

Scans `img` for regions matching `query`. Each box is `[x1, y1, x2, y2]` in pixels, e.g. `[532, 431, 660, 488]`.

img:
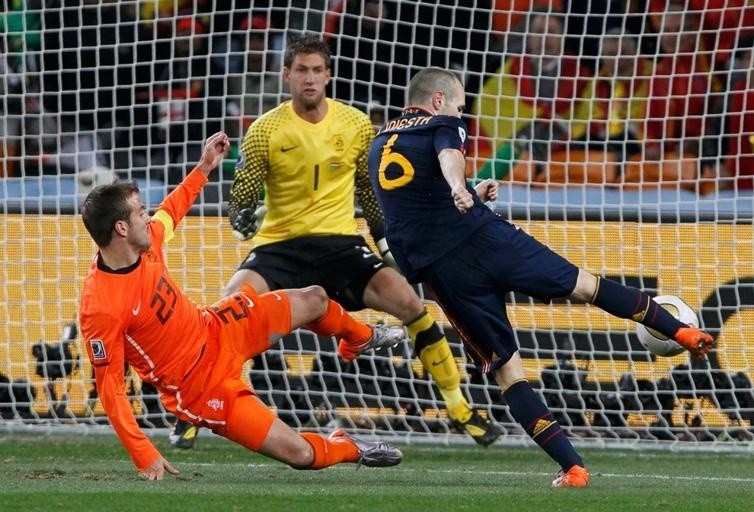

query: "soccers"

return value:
[635, 295, 700, 357]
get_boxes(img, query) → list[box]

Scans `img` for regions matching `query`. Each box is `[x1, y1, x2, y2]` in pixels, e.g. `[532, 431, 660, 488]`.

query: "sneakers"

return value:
[168, 418, 198, 448]
[451, 410, 504, 448]
[675, 325, 714, 359]
[328, 429, 403, 471]
[338, 324, 405, 361]
[552, 465, 590, 487]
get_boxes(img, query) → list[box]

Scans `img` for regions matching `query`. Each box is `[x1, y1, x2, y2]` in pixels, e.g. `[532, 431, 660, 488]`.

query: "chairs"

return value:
[461, 148, 730, 195]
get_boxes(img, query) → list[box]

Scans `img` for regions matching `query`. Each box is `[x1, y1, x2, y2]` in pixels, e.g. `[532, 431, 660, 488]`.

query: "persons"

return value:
[167, 32, 505, 451]
[1, 0, 754, 209]
[366, 66, 720, 487]
[80, 130, 409, 485]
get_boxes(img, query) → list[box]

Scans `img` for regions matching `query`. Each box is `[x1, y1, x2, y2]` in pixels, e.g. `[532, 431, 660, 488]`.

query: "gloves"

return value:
[232, 206, 269, 241]
[376, 236, 403, 274]
